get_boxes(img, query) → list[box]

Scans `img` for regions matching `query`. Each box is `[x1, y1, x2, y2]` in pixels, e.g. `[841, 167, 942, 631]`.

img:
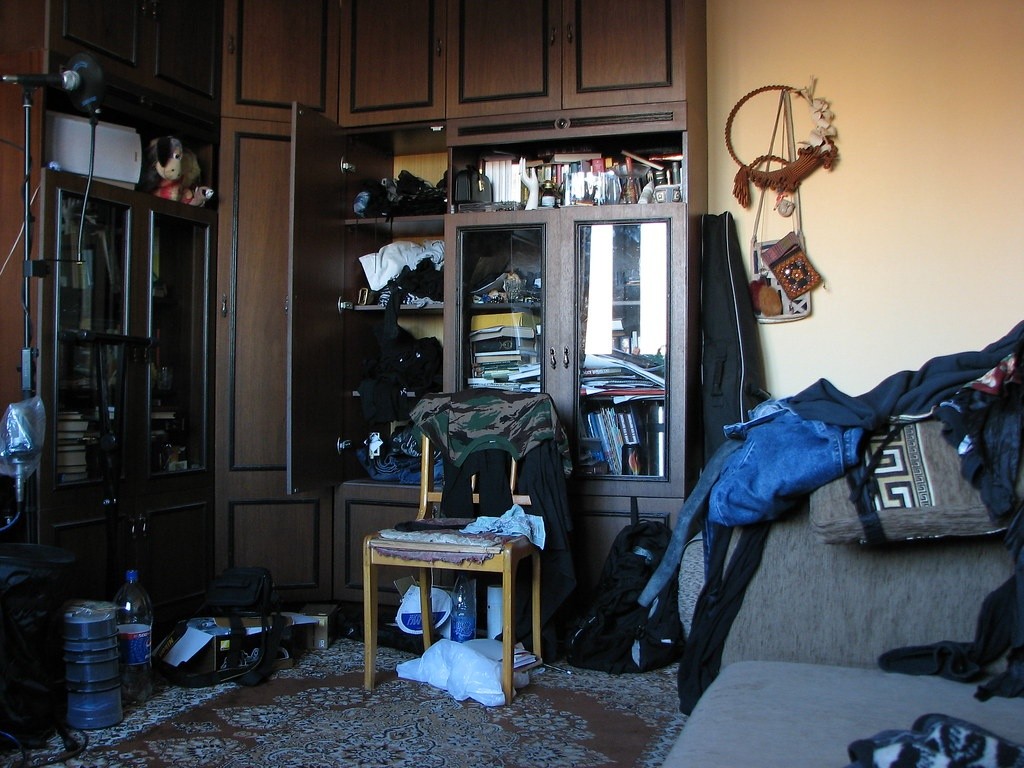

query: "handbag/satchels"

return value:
[204, 566, 275, 615]
[748, 229, 811, 324]
[760, 231, 822, 300]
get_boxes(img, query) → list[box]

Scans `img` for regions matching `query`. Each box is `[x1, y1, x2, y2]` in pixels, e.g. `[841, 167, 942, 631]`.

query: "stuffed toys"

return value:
[153, 146, 200, 205]
[135, 135, 182, 192]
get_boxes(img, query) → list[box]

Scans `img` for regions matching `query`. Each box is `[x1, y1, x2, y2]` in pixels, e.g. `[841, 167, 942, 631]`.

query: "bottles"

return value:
[450, 569, 475, 645]
[624, 157, 640, 205]
[636, 180, 654, 204]
[541, 182, 555, 208]
[114, 571, 156, 705]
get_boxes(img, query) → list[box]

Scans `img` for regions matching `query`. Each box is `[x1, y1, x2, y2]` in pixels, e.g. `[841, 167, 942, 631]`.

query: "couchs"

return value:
[656, 506, 1024, 768]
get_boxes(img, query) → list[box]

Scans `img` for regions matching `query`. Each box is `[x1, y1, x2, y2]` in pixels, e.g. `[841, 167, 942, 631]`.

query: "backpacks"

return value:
[565, 516, 687, 674]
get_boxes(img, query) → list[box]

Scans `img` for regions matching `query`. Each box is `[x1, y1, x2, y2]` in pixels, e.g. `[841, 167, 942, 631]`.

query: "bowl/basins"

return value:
[655, 185, 681, 205]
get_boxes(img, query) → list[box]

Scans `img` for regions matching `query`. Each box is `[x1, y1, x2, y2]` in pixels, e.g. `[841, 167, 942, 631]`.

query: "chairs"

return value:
[363, 391, 551, 712]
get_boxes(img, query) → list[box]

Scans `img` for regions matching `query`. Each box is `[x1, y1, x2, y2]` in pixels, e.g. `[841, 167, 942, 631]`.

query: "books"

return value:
[469, 325, 540, 392]
[578, 348, 664, 396]
[93, 412, 114, 420]
[67, 342, 117, 389]
[56, 410, 88, 481]
[151, 411, 176, 418]
[477, 145, 681, 207]
[497, 642, 542, 671]
[59, 249, 119, 334]
[92, 405, 114, 412]
[579, 403, 646, 475]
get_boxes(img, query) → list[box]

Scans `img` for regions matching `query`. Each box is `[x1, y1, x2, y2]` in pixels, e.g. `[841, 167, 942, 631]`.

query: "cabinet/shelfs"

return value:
[30, 0, 709, 663]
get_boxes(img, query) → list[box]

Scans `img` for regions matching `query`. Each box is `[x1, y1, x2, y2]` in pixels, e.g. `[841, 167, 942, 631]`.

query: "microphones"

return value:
[0, 70, 82, 92]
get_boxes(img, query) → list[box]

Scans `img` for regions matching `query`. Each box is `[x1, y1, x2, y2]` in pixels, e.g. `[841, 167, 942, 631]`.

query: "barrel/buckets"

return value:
[62, 598, 124, 728]
